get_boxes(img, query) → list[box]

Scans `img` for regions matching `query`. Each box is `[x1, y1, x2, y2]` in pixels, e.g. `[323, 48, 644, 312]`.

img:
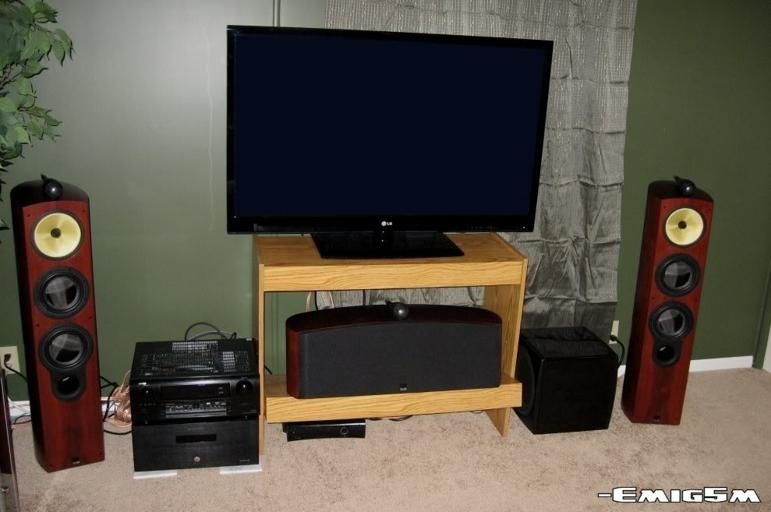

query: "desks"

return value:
[246, 233, 530, 454]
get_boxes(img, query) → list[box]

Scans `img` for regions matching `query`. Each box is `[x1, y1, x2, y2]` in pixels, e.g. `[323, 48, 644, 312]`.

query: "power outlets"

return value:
[609, 319, 620, 344]
[1, 346, 21, 375]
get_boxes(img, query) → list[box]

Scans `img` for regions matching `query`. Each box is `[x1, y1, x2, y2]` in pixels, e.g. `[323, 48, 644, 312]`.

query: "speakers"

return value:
[512, 325, 619, 435]
[284, 303, 502, 400]
[9, 180, 105, 475]
[129, 416, 261, 472]
[620, 180, 715, 426]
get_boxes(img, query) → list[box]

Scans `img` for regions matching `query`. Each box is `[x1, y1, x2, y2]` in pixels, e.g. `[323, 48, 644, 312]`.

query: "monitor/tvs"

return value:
[226, 24, 554, 259]
